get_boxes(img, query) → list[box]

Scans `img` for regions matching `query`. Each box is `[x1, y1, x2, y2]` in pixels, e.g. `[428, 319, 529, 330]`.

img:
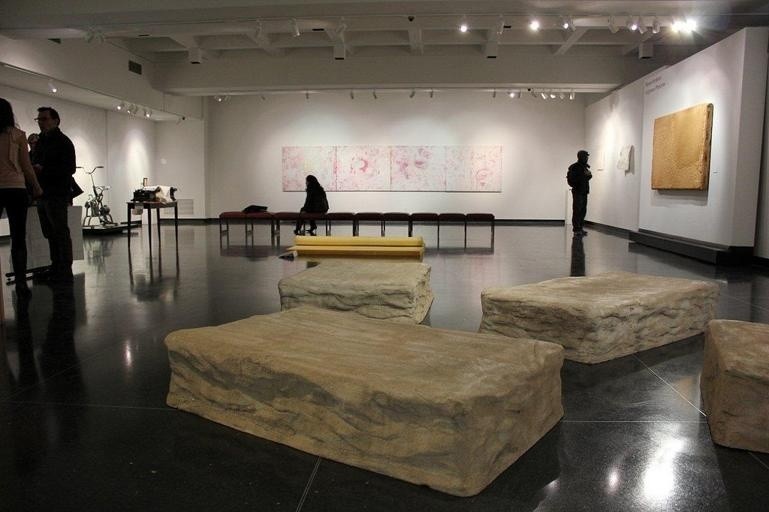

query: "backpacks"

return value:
[566, 162, 585, 187]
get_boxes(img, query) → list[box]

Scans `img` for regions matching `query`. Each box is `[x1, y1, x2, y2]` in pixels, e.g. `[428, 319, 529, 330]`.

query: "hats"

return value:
[577, 150, 589, 160]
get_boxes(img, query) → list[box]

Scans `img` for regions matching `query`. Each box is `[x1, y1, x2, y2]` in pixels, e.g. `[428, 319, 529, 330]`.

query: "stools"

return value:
[218, 212, 496, 253]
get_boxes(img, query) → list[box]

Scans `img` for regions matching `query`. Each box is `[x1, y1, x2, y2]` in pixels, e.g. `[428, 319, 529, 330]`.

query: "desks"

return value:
[127, 198, 180, 248]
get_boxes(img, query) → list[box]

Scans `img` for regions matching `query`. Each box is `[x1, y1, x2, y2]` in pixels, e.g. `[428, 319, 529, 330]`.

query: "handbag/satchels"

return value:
[66, 176, 84, 202]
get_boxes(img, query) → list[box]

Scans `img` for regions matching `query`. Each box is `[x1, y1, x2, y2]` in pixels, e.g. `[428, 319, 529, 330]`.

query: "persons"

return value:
[0, 295, 49, 477]
[568, 150, 593, 235]
[1, 98, 43, 296]
[30, 109, 76, 280]
[292, 175, 329, 234]
[27, 133, 41, 163]
[34, 280, 89, 467]
[570, 233, 587, 277]
